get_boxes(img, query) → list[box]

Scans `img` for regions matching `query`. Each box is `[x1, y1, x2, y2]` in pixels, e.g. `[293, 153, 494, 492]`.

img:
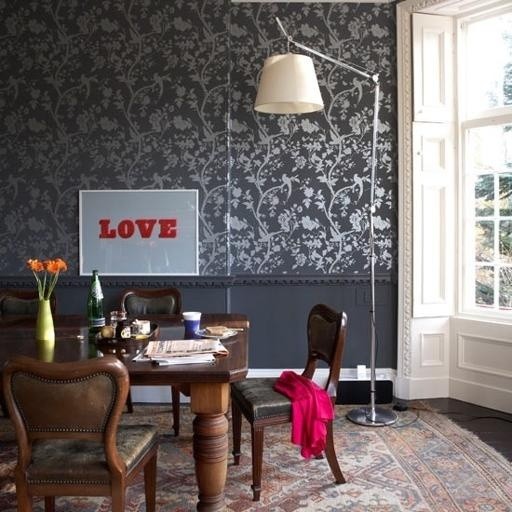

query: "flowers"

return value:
[26, 256, 69, 303]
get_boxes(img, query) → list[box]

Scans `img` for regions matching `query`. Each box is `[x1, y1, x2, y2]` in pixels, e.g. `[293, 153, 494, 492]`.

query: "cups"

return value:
[181, 311, 202, 334]
[132, 319, 151, 334]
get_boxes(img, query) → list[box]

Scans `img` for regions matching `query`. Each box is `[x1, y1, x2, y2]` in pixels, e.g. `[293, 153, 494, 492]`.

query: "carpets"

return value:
[0, 398, 510, 512]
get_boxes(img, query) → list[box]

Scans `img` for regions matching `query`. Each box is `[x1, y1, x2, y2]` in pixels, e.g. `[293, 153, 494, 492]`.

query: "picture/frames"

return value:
[77, 187, 201, 278]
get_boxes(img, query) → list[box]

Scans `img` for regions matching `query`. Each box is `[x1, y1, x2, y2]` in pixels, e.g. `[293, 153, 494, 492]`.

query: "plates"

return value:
[196, 329, 238, 340]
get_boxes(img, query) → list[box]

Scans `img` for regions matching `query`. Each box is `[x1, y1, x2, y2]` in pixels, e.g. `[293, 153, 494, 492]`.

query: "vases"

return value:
[33, 298, 56, 341]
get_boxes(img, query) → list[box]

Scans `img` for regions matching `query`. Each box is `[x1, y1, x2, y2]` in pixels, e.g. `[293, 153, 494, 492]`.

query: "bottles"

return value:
[88, 270, 105, 327]
[109, 310, 131, 340]
[87, 330, 104, 358]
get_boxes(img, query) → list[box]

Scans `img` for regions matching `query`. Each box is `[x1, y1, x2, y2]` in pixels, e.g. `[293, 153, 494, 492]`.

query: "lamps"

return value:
[253, 14, 399, 430]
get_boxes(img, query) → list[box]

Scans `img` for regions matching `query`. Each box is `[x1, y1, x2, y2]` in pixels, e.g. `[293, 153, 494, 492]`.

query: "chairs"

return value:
[114, 286, 183, 437]
[0, 287, 57, 317]
[227, 302, 350, 503]
[0, 350, 161, 511]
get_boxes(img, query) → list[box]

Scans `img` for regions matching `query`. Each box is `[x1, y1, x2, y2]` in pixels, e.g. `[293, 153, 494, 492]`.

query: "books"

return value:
[130, 338, 229, 367]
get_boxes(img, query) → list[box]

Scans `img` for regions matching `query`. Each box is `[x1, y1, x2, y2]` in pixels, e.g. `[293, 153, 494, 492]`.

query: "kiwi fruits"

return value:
[101, 325, 114, 338]
